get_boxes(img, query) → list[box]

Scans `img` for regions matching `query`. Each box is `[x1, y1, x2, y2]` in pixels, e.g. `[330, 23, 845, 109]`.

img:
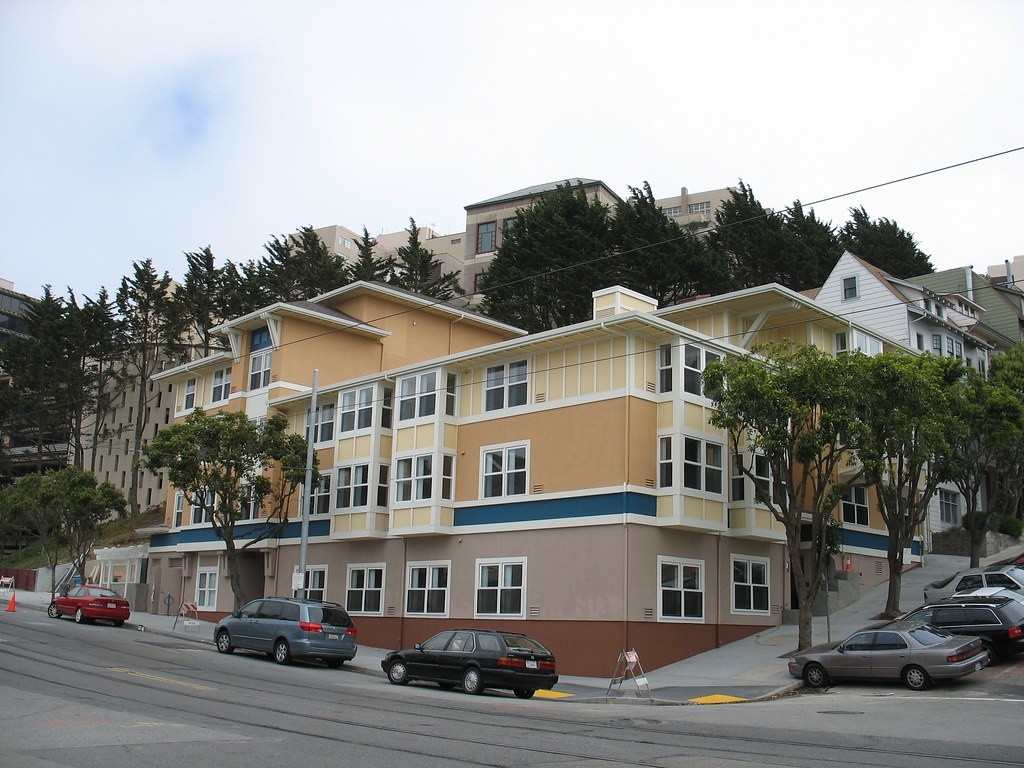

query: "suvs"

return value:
[890, 597, 1024, 666]
[213, 596, 358, 669]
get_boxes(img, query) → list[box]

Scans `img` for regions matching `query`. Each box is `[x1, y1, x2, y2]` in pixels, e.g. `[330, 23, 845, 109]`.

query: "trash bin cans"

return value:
[60, 584, 70, 597]
[74, 574, 87, 587]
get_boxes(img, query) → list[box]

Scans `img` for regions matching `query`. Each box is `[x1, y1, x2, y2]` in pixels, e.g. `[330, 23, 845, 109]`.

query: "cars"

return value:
[788, 620, 989, 691]
[949, 586, 1024, 607]
[47, 586, 130, 627]
[923, 565, 1024, 603]
[380, 630, 559, 699]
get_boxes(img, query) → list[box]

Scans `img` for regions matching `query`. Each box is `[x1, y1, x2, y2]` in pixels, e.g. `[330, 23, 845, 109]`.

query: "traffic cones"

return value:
[5, 593, 17, 612]
[845, 556, 852, 570]
